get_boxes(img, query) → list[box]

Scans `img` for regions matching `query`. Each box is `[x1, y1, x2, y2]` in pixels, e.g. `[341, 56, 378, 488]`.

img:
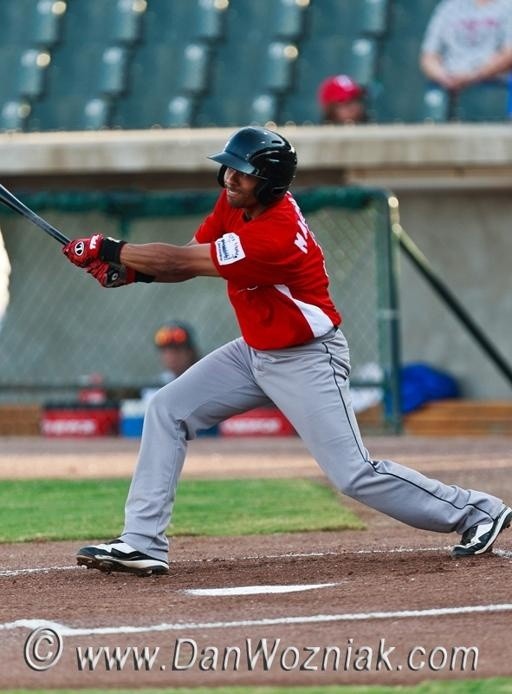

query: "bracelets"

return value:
[100, 233, 126, 262]
[134, 270, 157, 283]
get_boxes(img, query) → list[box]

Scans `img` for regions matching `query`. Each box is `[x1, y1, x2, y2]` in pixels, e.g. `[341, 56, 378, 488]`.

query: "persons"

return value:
[154, 318, 200, 384]
[317, 74, 371, 124]
[418, 0, 512, 121]
[61, 124, 512, 576]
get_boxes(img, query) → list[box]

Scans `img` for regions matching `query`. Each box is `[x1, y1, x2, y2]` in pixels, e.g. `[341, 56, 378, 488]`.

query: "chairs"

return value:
[0, 0, 512, 135]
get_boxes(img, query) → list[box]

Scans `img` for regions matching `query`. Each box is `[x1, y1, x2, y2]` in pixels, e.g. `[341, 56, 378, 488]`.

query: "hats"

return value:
[155, 321, 193, 348]
[321, 74, 360, 105]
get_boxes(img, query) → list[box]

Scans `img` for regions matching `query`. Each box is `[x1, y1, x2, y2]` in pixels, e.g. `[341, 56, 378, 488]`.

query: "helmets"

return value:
[209, 129, 296, 204]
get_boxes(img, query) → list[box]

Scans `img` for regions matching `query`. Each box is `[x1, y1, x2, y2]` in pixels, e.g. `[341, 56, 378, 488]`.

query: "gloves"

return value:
[87, 258, 138, 289]
[64, 233, 103, 266]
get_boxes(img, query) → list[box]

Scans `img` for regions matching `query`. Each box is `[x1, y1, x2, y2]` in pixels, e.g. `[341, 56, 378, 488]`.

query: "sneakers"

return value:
[75, 538, 170, 576]
[451, 503, 512, 558]
